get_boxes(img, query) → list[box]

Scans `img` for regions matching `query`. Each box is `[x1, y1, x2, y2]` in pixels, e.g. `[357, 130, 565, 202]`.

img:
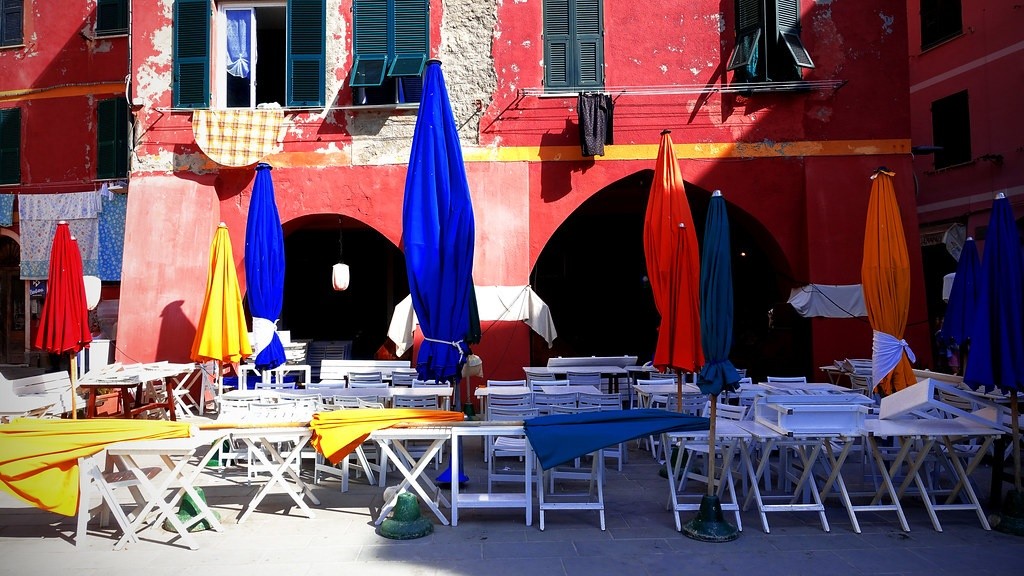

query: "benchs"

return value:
[13, 371, 104, 418]
[318, 359, 411, 388]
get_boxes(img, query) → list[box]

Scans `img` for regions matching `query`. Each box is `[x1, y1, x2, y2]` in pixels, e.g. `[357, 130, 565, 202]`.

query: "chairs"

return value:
[71, 340, 1024, 548]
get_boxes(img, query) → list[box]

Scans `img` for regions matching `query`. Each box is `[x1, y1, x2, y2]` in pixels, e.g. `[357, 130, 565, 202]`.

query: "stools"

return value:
[879, 377, 1021, 434]
[754, 396, 875, 437]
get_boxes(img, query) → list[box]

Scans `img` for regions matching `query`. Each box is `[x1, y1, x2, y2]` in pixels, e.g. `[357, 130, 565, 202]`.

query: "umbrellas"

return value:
[963, 191, 1023, 500]
[941, 235, 982, 377]
[697, 190, 742, 494]
[402, 58, 481, 414]
[861, 167, 916, 475]
[191, 222, 252, 397]
[643, 129, 706, 441]
[35, 221, 92, 419]
[243, 161, 287, 385]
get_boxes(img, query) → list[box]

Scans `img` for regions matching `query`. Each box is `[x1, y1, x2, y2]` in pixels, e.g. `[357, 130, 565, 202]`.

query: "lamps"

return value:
[331, 220, 351, 292]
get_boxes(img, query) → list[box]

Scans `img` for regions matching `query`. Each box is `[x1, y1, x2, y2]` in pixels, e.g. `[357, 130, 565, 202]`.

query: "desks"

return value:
[685, 382, 769, 420]
[452, 426, 532, 527]
[474, 386, 531, 462]
[758, 382, 851, 395]
[371, 425, 453, 527]
[238, 364, 312, 390]
[632, 383, 701, 451]
[79, 375, 178, 422]
[215, 387, 454, 447]
[101, 432, 225, 552]
[819, 365, 846, 385]
[624, 365, 691, 385]
[662, 422, 752, 532]
[731, 421, 840, 533]
[522, 367, 628, 399]
[232, 427, 320, 525]
[985, 394, 1024, 468]
[820, 419, 1006, 534]
[540, 385, 604, 415]
